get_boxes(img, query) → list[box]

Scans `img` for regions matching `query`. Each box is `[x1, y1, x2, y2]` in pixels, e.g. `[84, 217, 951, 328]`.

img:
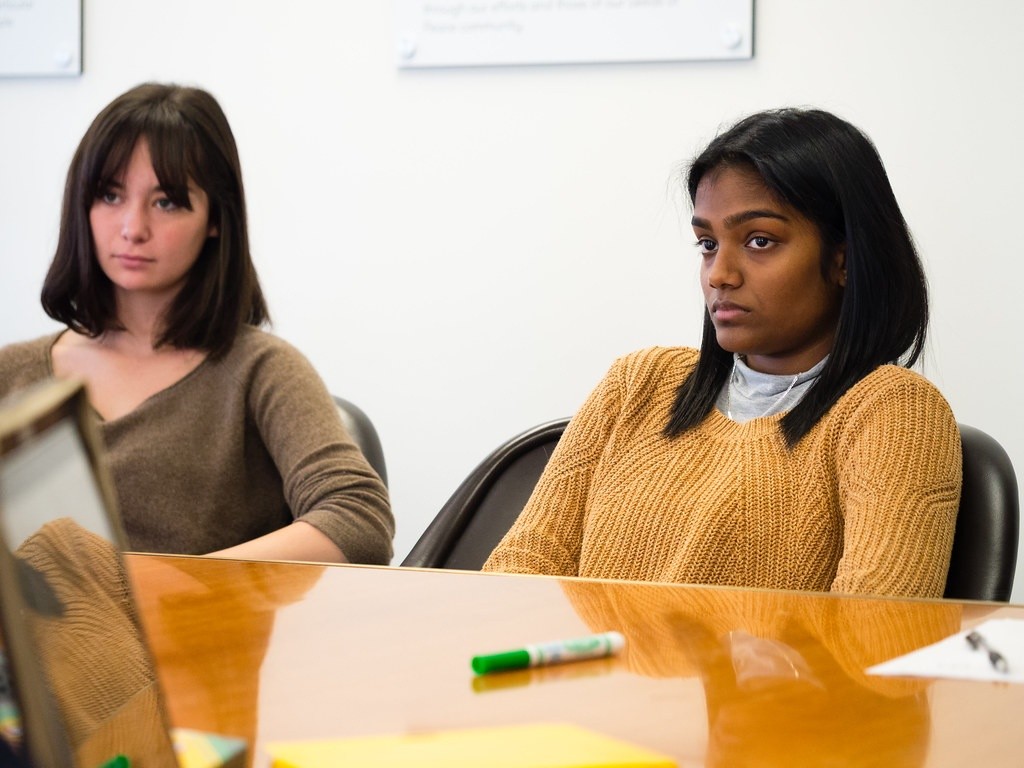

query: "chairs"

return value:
[940, 422, 1020, 602]
[398, 417, 574, 576]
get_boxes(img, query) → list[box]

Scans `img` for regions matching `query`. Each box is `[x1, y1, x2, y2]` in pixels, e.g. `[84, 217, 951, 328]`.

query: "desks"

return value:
[121, 552, 1024, 768]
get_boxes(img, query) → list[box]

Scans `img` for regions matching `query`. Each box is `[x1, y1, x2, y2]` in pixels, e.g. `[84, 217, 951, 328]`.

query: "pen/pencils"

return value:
[965, 624, 1009, 676]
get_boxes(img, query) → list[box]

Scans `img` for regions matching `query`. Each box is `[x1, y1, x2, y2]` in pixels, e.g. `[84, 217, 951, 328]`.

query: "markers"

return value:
[471, 631, 626, 676]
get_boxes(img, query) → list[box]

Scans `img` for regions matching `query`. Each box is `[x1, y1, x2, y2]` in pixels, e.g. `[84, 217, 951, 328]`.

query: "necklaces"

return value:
[728, 356, 803, 420]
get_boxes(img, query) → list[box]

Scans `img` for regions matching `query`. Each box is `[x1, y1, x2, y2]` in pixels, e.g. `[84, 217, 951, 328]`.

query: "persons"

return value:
[0, 82, 397, 566]
[479, 110, 962, 600]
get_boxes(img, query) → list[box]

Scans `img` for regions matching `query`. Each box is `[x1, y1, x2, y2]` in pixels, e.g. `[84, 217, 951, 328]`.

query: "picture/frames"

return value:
[0, 379, 182, 768]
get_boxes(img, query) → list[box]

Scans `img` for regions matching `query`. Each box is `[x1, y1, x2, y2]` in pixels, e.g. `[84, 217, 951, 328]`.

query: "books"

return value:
[0, 377, 182, 767]
